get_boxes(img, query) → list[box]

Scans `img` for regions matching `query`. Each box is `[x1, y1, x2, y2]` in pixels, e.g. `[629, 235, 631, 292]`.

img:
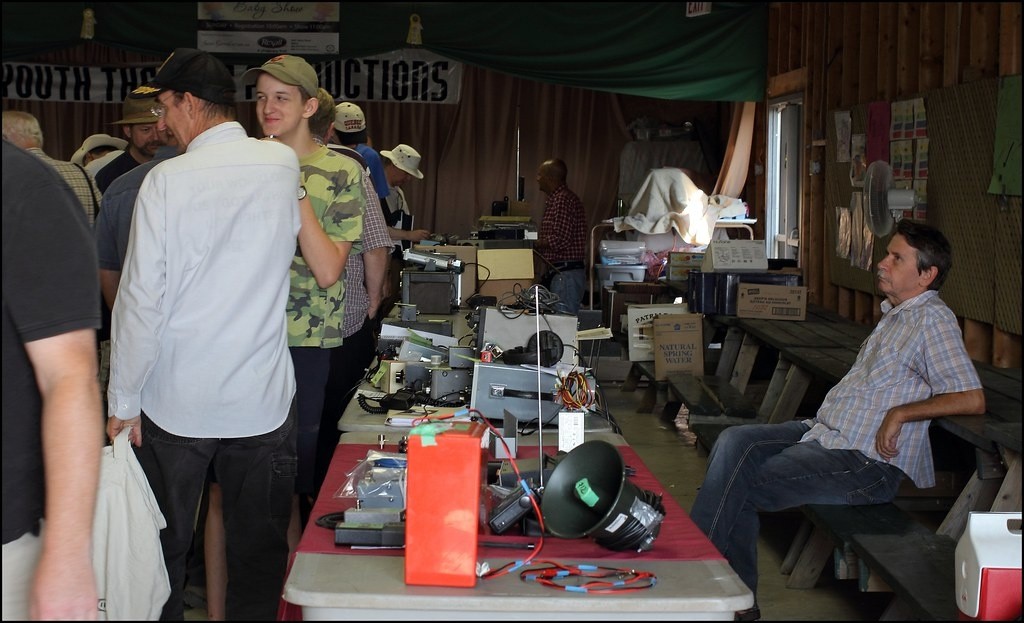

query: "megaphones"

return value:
[542, 438, 665, 551]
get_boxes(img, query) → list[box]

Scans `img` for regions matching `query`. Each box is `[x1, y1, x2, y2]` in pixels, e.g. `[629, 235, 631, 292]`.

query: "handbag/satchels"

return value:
[91, 423, 171, 622]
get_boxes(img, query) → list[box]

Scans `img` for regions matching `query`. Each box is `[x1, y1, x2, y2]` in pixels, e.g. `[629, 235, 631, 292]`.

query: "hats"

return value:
[241, 54, 318, 100]
[380, 143, 425, 179]
[333, 102, 365, 133]
[102, 85, 161, 124]
[129, 47, 236, 108]
[71, 134, 131, 166]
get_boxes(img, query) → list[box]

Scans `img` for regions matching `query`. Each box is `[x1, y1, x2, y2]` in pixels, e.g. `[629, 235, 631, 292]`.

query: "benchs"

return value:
[625, 353, 969, 623]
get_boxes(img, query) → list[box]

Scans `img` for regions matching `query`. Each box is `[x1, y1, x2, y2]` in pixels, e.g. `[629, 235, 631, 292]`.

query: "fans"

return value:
[861, 160, 917, 240]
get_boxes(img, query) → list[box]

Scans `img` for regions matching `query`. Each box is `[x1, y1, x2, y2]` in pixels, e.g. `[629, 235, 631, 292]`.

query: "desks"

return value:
[283, 431, 754, 623]
[336, 353, 614, 431]
[718, 312, 1024, 548]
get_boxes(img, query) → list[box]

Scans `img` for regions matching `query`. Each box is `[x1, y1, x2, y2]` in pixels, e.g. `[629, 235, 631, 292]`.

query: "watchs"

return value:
[296, 186, 307, 200]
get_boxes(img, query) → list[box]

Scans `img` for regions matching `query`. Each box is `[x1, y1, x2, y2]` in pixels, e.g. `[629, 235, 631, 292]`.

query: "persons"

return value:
[329, 102, 390, 198]
[380, 144, 430, 298]
[71, 134, 128, 175]
[309, 88, 396, 501]
[688, 218, 985, 621]
[2, 139, 106, 621]
[94, 127, 179, 313]
[2, 110, 103, 229]
[106, 47, 302, 621]
[536, 158, 586, 314]
[239, 54, 367, 531]
[95, 95, 165, 197]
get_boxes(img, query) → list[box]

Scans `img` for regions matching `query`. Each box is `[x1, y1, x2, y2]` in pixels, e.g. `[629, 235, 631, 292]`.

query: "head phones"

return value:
[502, 330, 564, 368]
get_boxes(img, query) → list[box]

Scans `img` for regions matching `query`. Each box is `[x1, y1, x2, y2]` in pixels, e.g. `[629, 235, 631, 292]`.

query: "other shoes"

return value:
[182, 584, 208, 609]
[735, 599, 760, 620]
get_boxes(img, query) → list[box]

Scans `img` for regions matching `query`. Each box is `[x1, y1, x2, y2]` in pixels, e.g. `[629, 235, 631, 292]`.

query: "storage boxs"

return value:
[477, 249, 534, 280]
[479, 281, 532, 307]
[595, 239, 807, 381]
[415, 244, 476, 306]
